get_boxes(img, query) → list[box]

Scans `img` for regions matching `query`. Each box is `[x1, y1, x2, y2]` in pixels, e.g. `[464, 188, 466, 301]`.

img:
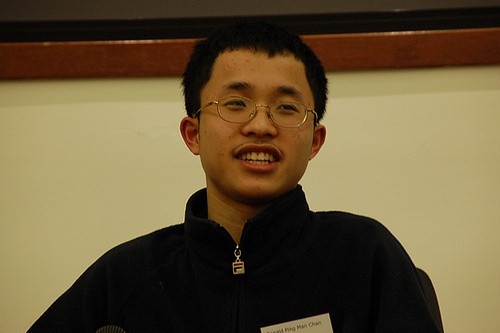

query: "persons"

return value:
[25, 20, 440, 332]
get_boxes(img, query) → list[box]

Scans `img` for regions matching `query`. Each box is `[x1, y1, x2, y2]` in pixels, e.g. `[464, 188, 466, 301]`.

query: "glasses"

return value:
[191, 96, 319, 129]
